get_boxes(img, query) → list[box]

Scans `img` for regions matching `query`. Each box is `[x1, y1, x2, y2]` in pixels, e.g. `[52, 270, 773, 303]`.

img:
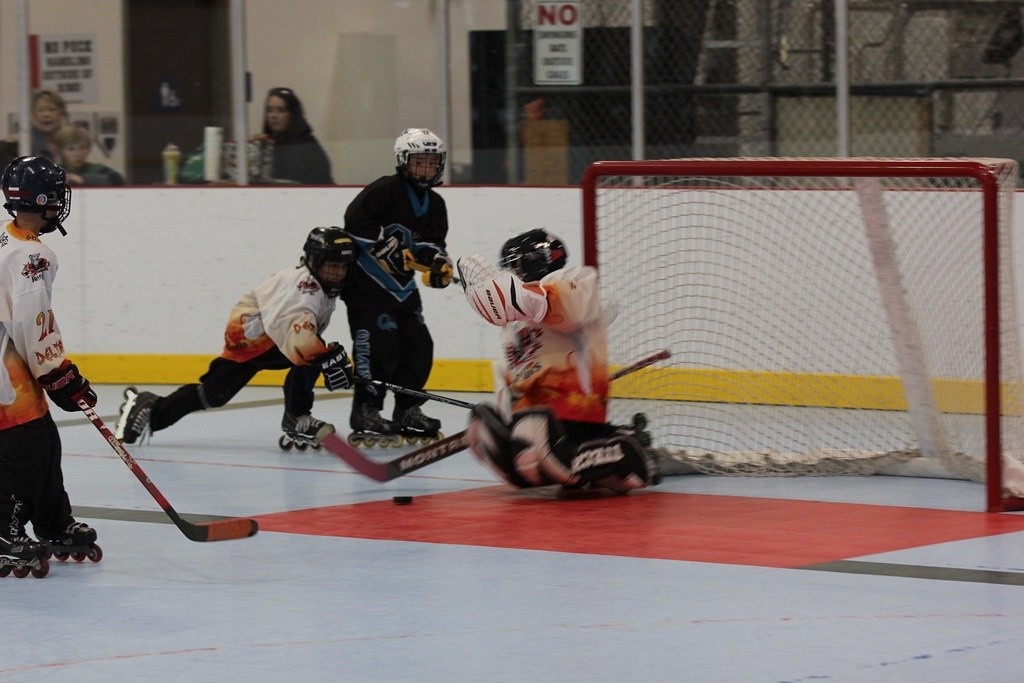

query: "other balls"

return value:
[393, 496, 412, 503]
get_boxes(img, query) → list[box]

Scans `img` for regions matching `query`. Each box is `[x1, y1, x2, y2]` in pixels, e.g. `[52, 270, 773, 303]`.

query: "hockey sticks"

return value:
[353, 374, 652, 447]
[369, 247, 463, 286]
[75, 396, 260, 543]
[316, 347, 673, 486]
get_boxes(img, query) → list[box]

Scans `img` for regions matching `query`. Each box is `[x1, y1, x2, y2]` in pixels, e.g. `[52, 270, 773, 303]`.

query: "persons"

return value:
[341, 128, 454, 448]
[456, 229, 672, 496]
[0, 156, 102, 579]
[248, 87, 336, 185]
[112, 226, 359, 443]
[0, 90, 127, 186]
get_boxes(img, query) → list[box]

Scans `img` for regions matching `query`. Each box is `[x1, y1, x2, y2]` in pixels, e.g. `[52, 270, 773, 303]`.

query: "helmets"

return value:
[2, 155, 72, 234]
[394, 128, 446, 188]
[499, 228, 568, 283]
[303, 227, 352, 294]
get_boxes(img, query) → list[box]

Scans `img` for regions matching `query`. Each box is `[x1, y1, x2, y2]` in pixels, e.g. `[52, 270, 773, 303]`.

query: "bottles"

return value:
[161, 150, 183, 187]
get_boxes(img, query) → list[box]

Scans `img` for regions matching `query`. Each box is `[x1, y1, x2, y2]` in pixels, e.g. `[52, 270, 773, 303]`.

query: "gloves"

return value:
[374, 236, 415, 278]
[309, 343, 354, 393]
[421, 253, 453, 289]
[36, 360, 98, 413]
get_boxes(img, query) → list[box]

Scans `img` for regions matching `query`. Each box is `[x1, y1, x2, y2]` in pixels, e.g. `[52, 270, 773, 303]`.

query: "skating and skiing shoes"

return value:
[278, 408, 336, 452]
[32, 517, 102, 563]
[0, 526, 53, 579]
[348, 402, 405, 449]
[113, 385, 163, 445]
[392, 395, 446, 445]
